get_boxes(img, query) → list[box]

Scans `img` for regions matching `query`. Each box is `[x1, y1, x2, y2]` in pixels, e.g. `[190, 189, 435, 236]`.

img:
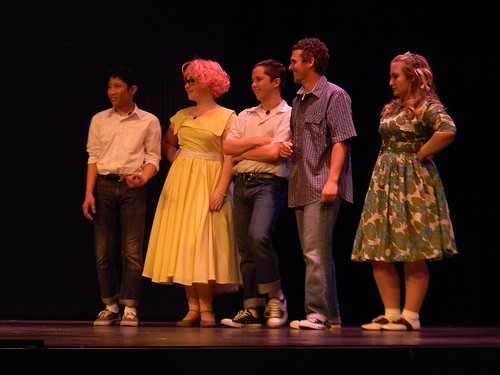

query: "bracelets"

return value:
[217, 190, 228, 196]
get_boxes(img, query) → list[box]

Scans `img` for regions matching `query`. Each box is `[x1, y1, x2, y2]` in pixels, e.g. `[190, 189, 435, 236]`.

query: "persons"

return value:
[220, 59, 294, 328]
[280, 38, 357, 330]
[141, 58, 243, 328]
[83, 73, 162, 327]
[351, 51, 457, 331]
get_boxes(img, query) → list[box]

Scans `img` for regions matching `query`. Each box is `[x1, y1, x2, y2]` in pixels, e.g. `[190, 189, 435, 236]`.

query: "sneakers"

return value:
[265, 297, 287, 327]
[94, 309, 121, 325]
[120, 306, 138, 325]
[290, 320, 341, 329]
[298, 318, 331, 329]
[221, 309, 262, 328]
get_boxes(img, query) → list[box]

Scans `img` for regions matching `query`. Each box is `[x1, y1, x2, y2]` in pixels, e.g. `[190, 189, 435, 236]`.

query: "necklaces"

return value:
[193, 111, 200, 119]
[260, 104, 278, 114]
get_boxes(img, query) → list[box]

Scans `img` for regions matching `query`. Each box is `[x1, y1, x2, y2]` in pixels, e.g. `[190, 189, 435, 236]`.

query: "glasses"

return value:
[184, 79, 195, 85]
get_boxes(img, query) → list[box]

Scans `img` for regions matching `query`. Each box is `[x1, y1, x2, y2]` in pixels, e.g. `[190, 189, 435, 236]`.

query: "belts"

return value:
[236, 173, 274, 179]
[99, 173, 127, 181]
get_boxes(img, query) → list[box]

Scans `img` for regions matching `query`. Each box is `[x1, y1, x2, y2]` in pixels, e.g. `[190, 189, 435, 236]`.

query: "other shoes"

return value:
[380, 315, 422, 330]
[362, 316, 393, 330]
[200, 311, 216, 327]
[176, 310, 200, 327]
